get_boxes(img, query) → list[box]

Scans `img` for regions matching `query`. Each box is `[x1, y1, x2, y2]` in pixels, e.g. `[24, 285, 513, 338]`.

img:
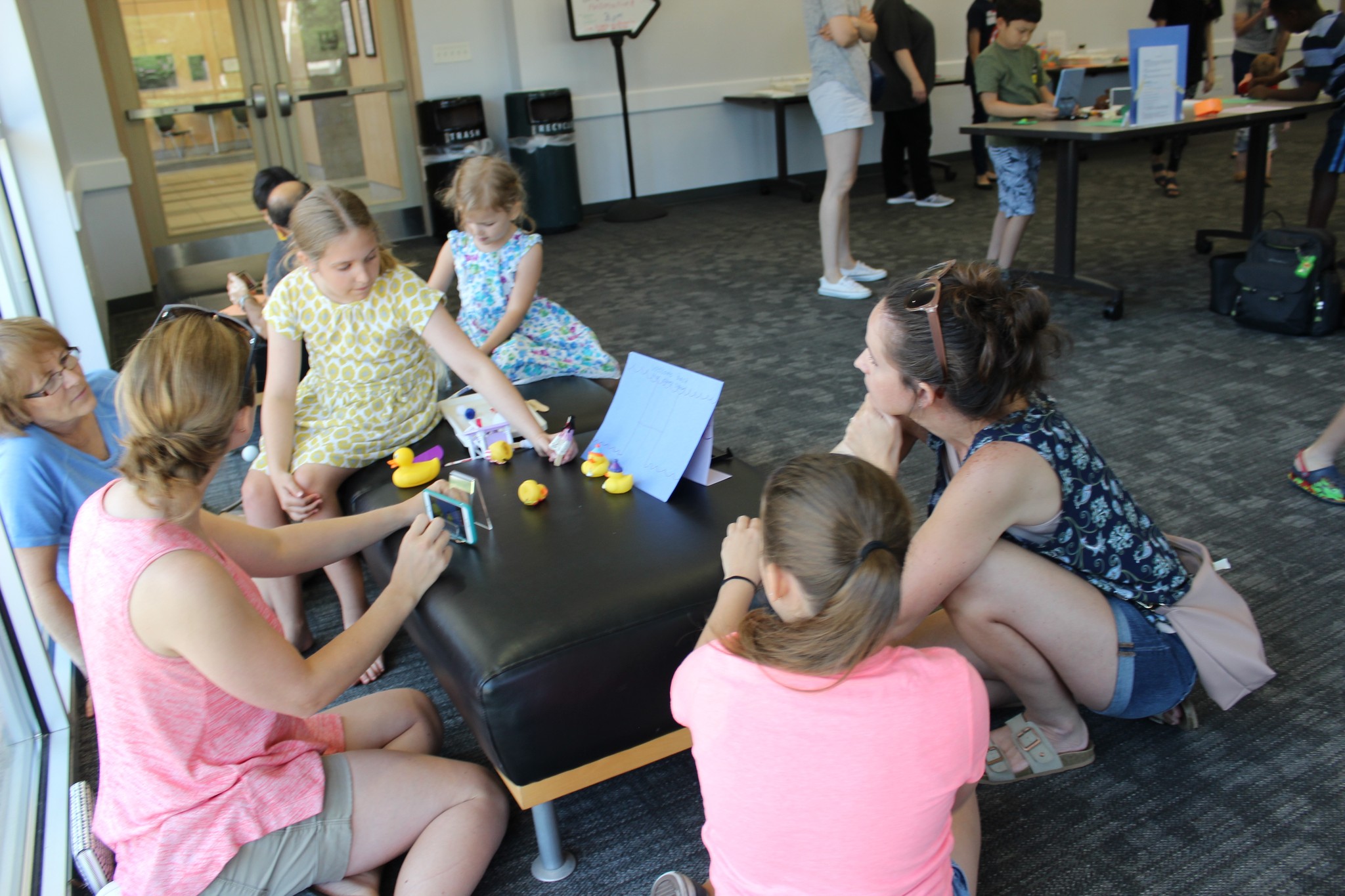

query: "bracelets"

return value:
[719, 576, 756, 594]
[852, 18, 861, 28]
[1207, 70, 1217, 76]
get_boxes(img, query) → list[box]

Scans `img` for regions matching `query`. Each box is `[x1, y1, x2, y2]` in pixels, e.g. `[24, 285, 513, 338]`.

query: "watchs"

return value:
[239, 295, 255, 309]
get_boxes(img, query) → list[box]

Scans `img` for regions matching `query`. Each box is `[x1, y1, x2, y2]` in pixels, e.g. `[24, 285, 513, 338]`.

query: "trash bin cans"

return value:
[505, 86, 585, 235]
[415, 94, 496, 245]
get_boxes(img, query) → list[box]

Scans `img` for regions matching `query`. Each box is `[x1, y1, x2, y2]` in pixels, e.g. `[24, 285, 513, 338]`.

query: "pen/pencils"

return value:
[445, 385, 472, 400]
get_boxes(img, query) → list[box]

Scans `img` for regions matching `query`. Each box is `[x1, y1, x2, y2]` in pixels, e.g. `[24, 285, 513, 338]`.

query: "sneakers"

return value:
[840, 260, 887, 282]
[817, 274, 872, 300]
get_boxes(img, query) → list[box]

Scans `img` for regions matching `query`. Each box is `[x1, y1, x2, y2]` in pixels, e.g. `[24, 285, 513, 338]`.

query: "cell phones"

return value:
[422, 489, 477, 545]
[1053, 67, 1086, 117]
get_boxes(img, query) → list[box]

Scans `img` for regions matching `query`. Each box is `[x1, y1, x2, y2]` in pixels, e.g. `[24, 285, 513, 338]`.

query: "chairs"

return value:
[156, 115, 200, 158]
[232, 107, 254, 150]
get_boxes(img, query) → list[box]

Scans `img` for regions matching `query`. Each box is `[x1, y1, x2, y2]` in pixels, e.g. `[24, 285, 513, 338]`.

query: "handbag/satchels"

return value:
[1155, 532, 1277, 712]
[1208, 211, 1286, 314]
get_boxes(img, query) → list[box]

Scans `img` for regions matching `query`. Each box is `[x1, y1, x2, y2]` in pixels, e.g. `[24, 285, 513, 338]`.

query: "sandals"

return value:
[1163, 178, 1180, 197]
[1152, 163, 1168, 187]
[1288, 451, 1345, 505]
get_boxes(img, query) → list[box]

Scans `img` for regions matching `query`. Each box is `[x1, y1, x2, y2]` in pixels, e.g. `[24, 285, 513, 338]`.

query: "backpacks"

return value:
[1231, 227, 1340, 338]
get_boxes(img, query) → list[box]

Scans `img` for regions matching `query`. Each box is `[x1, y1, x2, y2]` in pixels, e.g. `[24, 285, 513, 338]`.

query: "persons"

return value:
[974, 0, 1079, 277]
[798, 0, 887, 300]
[964, 0, 1001, 192]
[426, 155, 620, 386]
[68, 304, 511, 896]
[1147, 0, 1224, 198]
[1231, 0, 1345, 230]
[829, 260, 1272, 786]
[651, 454, 988, 895]
[0, 318, 133, 717]
[213, 167, 313, 409]
[1288, 404, 1345, 505]
[242, 184, 577, 685]
[870, 0, 955, 207]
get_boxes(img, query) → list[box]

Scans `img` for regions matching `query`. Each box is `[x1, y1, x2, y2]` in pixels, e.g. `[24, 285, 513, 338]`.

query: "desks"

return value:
[724, 62, 1334, 321]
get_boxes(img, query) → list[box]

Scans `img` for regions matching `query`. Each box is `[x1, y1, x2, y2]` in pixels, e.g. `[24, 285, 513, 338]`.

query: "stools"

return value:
[336, 374, 767, 883]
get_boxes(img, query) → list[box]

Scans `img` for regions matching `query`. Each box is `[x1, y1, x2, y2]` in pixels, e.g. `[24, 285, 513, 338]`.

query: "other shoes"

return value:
[651, 870, 706, 896]
[887, 191, 915, 203]
[975, 176, 994, 189]
[915, 193, 955, 208]
[1264, 174, 1271, 186]
[1235, 170, 1247, 181]
[1232, 151, 1239, 157]
[985, 171, 997, 181]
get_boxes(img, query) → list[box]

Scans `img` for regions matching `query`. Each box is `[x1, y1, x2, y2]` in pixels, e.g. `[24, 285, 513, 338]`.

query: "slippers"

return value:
[1146, 698, 1198, 731]
[978, 713, 1095, 786]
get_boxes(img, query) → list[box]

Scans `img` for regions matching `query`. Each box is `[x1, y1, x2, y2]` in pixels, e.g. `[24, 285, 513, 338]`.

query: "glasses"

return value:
[22, 347, 80, 398]
[149, 305, 260, 395]
[904, 259, 956, 398]
